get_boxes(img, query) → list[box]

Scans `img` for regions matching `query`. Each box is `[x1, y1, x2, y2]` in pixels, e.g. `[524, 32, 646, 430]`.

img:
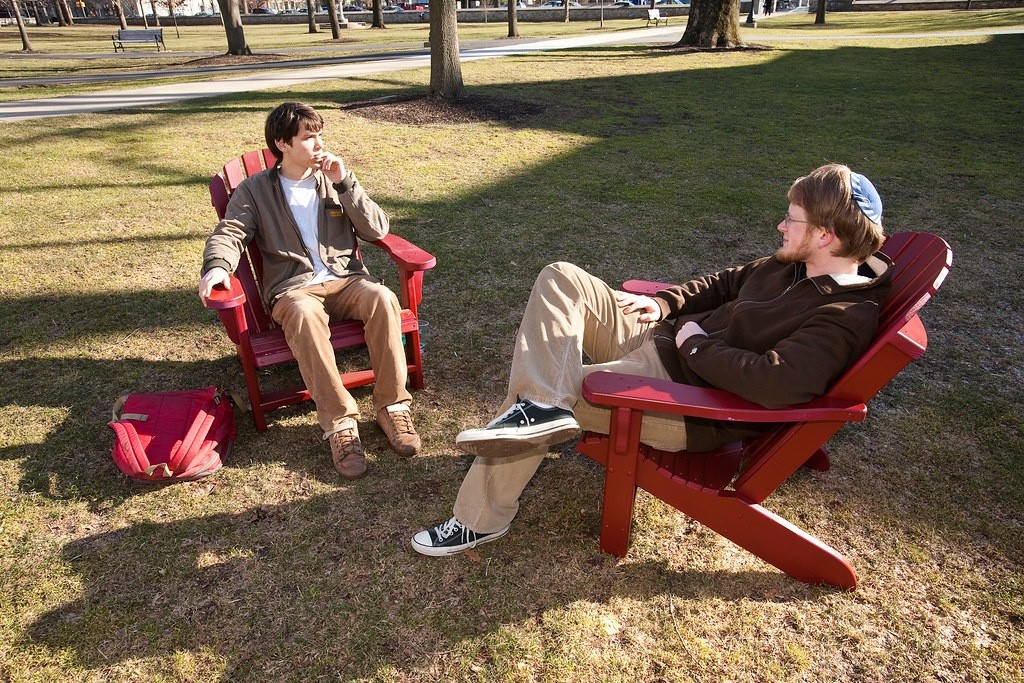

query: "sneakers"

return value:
[456, 393, 581, 459]
[411, 515, 512, 556]
[376, 403, 422, 457]
[328, 424, 369, 480]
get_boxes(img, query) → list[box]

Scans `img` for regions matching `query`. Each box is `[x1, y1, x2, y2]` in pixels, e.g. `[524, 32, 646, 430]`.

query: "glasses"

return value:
[783, 212, 808, 225]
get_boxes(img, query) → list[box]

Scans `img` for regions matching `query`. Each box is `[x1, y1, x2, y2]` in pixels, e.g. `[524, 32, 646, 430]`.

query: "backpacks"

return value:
[104, 386, 247, 482]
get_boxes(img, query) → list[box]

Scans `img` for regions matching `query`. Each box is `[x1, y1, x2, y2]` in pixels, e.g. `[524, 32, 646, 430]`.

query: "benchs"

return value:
[112, 28, 167, 52]
[646, 8, 669, 27]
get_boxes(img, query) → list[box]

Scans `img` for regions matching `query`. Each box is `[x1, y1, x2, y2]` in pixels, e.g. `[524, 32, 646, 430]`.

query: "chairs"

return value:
[200, 147, 436, 433]
[569, 226, 952, 592]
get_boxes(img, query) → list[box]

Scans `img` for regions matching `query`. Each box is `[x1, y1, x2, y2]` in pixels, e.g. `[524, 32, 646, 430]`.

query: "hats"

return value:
[850, 171, 882, 225]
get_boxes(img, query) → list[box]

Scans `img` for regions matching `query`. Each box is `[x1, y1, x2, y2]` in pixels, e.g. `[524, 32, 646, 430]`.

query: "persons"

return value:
[409, 160, 897, 557]
[198, 100, 421, 478]
